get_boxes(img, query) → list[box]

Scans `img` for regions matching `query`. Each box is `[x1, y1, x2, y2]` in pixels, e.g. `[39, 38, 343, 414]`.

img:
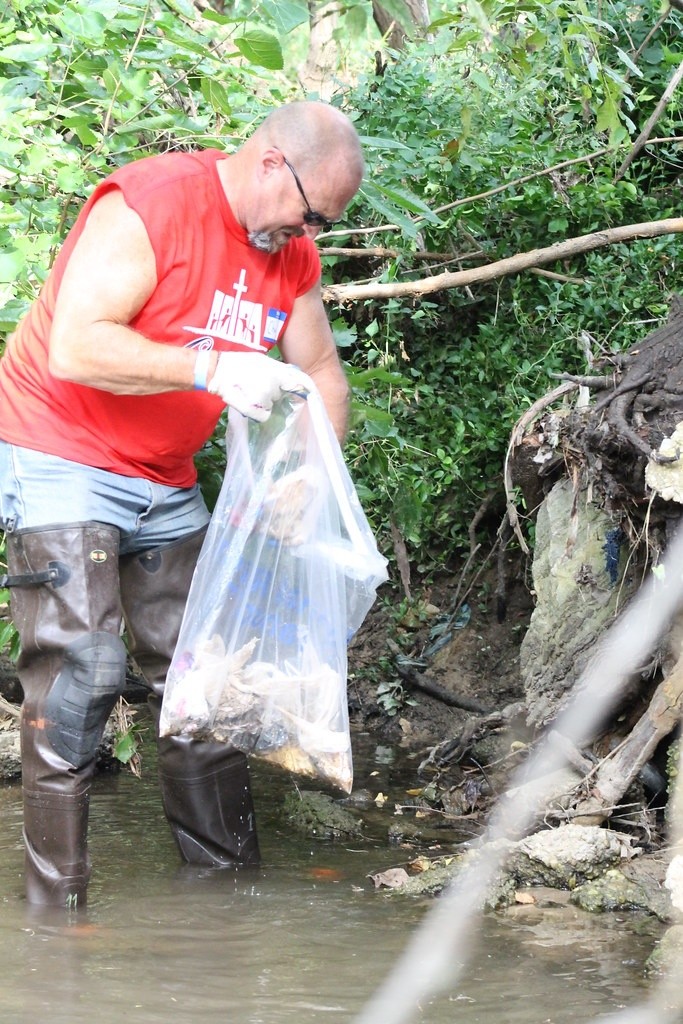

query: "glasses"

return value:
[280, 154, 337, 233]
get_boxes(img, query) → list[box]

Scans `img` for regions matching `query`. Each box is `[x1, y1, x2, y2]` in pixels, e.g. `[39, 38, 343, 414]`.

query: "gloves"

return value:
[208, 351, 301, 424]
[263, 467, 326, 545]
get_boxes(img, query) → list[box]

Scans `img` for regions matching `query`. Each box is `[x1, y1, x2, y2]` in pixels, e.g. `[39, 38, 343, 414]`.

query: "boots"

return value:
[119, 525, 339, 888]
[5, 522, 126, 942]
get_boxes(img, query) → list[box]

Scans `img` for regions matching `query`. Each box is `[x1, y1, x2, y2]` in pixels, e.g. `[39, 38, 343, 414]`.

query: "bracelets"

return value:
[194, 351, 209, 389]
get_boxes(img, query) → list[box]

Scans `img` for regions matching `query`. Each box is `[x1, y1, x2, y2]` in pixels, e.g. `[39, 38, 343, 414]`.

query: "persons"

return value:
[1, 100, 364, 911]
